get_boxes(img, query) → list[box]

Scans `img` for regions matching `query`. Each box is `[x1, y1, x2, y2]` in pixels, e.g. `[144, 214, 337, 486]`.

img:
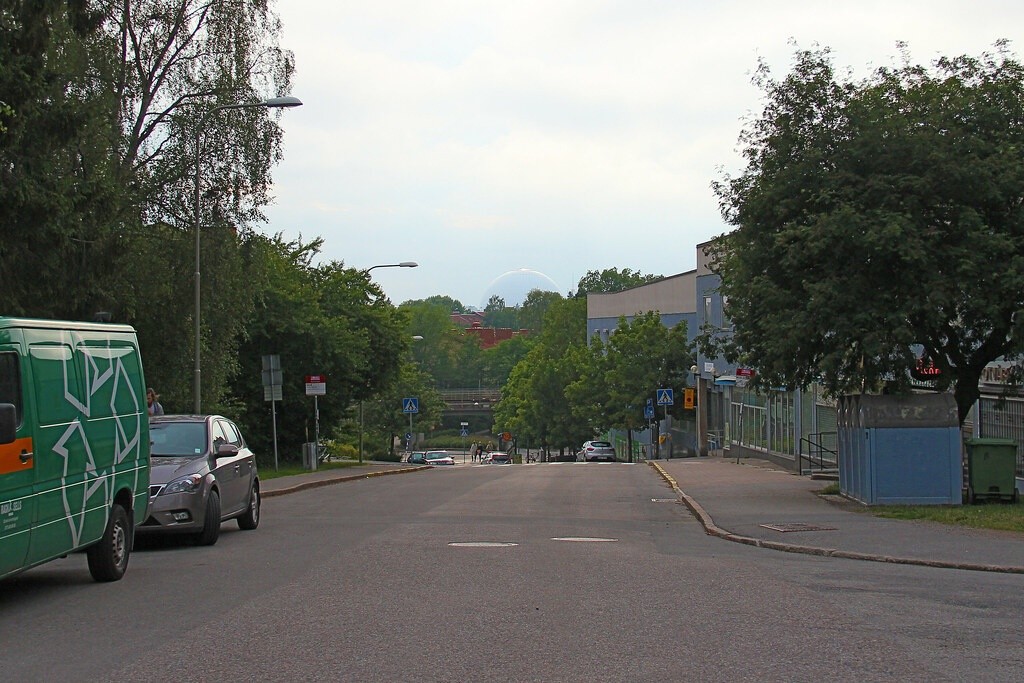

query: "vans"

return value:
[0, 312, 151, 582]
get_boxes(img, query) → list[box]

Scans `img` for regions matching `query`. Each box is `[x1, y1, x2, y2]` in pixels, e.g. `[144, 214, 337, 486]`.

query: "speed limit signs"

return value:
[503, 431, 511, 441]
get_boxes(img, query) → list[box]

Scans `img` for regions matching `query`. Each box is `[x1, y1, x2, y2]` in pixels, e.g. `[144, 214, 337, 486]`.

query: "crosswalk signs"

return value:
[459, 429, 468, 437]
[656, 389, 673, 406]
[402, 398, 418, 414]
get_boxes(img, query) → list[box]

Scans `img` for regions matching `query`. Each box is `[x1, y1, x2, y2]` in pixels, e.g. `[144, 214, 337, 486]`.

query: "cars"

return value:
[575, 441, 616, 463]
[420, 450, 456, 466]
[479, 451, 511, 464]
[400, 451, 425, 464]
[134, 414, 262, 549]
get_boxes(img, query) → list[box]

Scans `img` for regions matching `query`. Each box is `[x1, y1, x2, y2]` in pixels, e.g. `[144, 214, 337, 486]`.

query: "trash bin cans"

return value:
[963, 437, 1020, 504]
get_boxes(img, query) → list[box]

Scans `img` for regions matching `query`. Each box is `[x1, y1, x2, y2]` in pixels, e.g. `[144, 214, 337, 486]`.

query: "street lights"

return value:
[389, 334, 424, 462]
[358, 260, 420, 463]
[193, 95, 304, 415]
[690, 365, 701, 458]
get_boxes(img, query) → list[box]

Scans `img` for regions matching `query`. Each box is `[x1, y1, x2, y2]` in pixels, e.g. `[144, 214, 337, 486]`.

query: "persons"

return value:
[486, 441, 493, 450]
[530, 452, 534, 463]
[471, 441, 477, 462]
[476, 442, 483, 462]
[146, 388, 163, 415]
